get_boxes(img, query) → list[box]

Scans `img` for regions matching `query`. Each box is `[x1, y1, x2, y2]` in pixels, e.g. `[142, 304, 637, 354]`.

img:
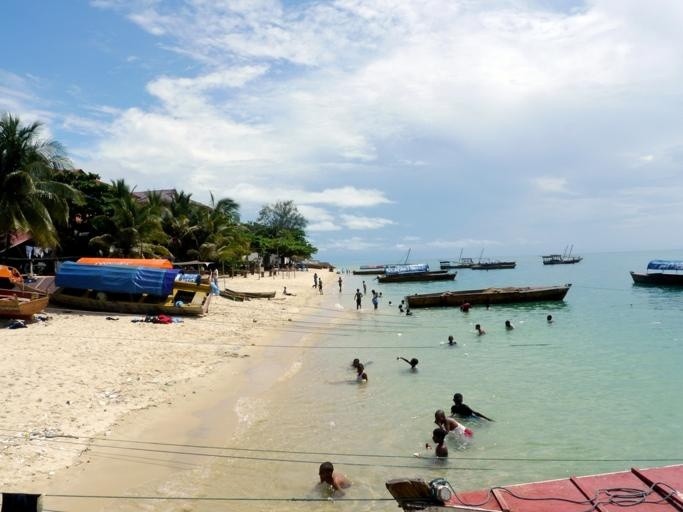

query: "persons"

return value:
[318, 461, 352, 498]
[445, 336, 456, 344]
[505, 320, 514, 330]
[425, 429, 448, 459]
[474, 324, 486, 336]
[434, 409, 473, 437]
[400, 356, 419, 373]
[203, 258, 279, 297]
[459, 298, 472, 312]
[355, 364, 368, 381]
[448, 393, 474, 422]
[352, 359, 362, 367]
[283, 285, 296, 297]
[546, 315, 554, 324]
[308, 268, 415, 317]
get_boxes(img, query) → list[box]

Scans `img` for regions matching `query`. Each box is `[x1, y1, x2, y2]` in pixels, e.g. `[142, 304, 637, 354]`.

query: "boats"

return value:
[0, 256, 221, 321]
[540, 242, 586, 265]
[629, 256, 682, 289]
[219, 288, 277, 304]
[385, 459, 682, 512]
[401, 279, 573, 307]
[351, 243, 518, 286]
